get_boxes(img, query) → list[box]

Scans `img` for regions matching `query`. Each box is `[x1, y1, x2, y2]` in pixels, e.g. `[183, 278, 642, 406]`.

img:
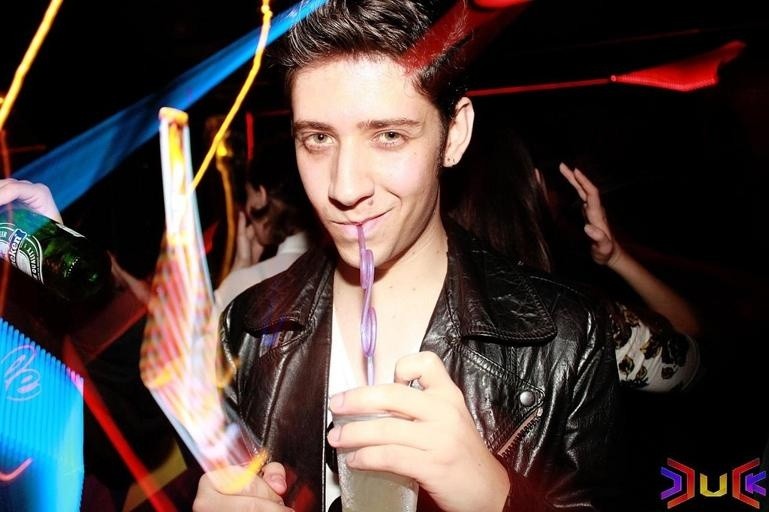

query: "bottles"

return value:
[1, 199, 113, 300]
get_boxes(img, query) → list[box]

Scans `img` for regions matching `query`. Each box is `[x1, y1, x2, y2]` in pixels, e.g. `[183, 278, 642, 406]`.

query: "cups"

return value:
[331, 411, 419, 512]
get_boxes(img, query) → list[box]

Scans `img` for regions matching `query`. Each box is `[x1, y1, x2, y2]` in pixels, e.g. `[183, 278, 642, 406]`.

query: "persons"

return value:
[214, 158, 309, 310]
[446, 130, 708, 394]
[191, 1, 632, 511]
[0, 177, 66, 225]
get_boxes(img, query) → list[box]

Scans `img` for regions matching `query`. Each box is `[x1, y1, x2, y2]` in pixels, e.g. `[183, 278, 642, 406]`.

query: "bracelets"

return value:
[608, 245, 621, 267]
